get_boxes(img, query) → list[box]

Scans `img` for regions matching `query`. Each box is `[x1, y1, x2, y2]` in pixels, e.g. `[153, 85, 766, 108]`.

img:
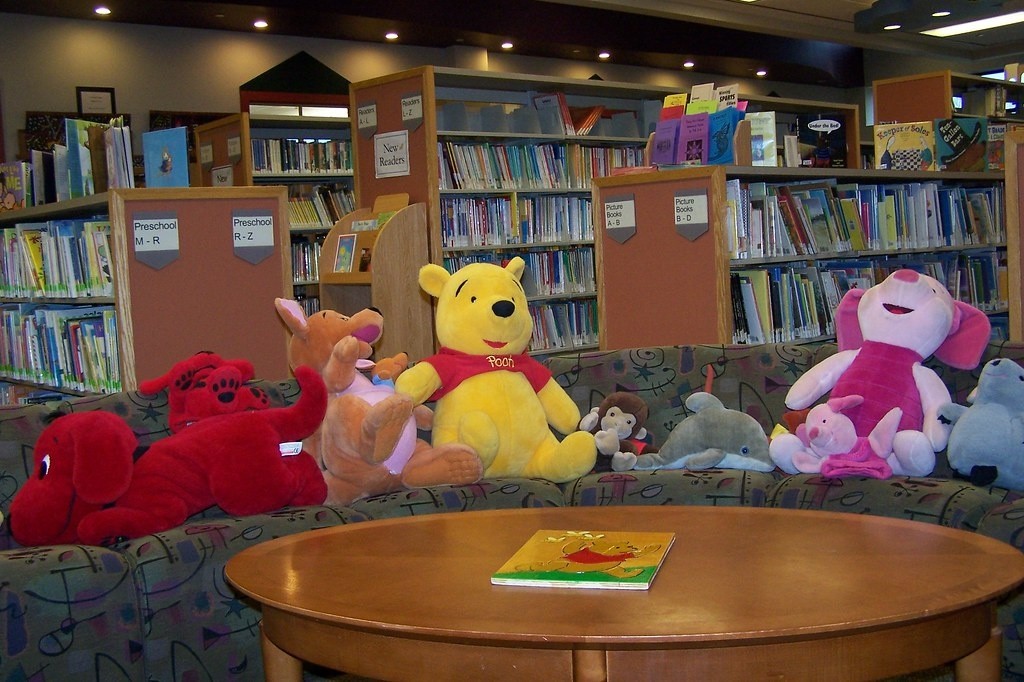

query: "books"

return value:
[0, 115, 191, 407]
[436, 82, 1010, 353]
[250, 136, 355, 318]
[490, 529, 677, 591]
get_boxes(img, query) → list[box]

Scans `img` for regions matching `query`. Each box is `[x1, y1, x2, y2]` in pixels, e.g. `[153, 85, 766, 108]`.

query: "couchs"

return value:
[0, 346, 1024, 681]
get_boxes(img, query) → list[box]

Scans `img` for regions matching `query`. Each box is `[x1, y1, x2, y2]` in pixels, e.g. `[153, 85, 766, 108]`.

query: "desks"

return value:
[224, 514, 1024, 682]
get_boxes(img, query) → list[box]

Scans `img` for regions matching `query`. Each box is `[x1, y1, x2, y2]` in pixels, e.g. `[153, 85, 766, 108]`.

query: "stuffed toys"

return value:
[9, 256, 1024, 548]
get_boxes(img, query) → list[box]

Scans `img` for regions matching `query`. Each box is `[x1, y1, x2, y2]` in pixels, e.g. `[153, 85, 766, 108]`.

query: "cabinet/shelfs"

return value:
[1, 67, 1023, 397]
